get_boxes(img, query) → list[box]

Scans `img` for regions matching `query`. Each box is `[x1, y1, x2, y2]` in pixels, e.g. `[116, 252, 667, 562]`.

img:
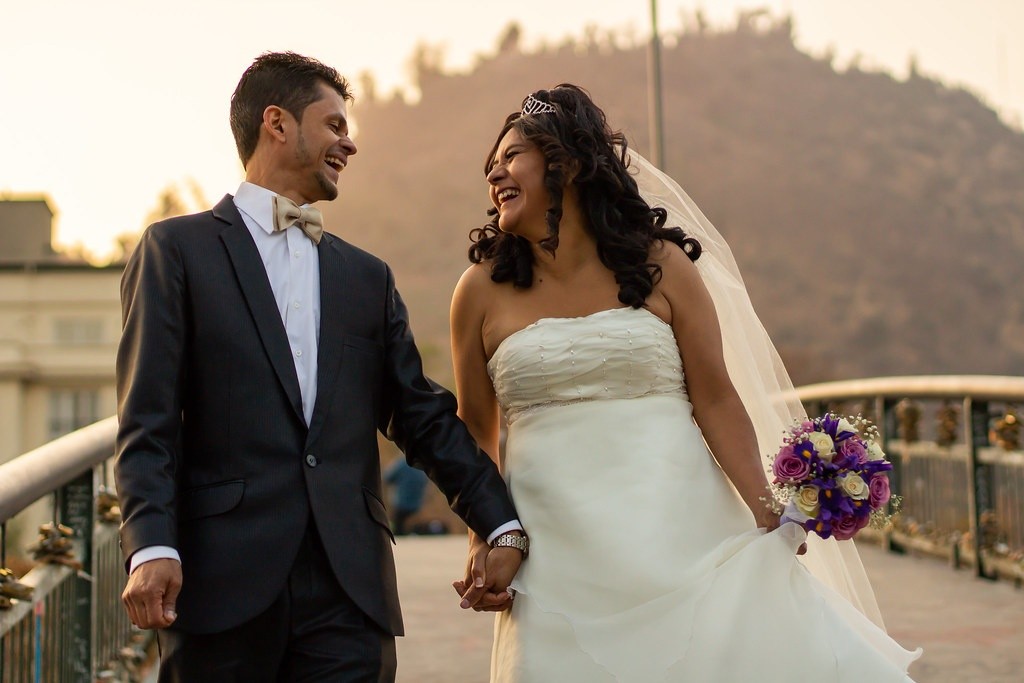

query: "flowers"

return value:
[759, 411, 905, 542]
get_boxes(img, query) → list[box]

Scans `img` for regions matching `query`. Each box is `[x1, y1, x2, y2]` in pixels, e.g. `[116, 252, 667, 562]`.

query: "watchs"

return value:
[492, 533, 531, 560]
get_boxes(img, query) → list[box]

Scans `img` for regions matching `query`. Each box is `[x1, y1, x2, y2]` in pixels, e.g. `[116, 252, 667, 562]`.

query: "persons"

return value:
[379, 452, 428, 536]
[450, 83, 926, 682]
[112, 50, 528, 683]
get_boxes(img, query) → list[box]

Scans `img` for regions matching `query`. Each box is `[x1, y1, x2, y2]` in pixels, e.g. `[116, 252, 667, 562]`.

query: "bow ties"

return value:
[272, 195, 324, 244]
